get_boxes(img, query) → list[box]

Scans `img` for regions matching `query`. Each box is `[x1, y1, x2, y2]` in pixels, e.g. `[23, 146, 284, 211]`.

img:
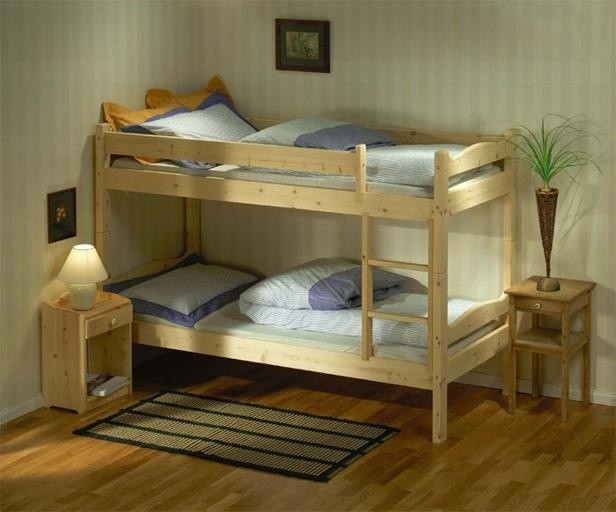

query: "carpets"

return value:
[73, 390, 400, 483]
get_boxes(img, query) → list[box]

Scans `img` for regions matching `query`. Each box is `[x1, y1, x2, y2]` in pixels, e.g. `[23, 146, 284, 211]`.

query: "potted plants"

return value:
[496, 113, 603, 292]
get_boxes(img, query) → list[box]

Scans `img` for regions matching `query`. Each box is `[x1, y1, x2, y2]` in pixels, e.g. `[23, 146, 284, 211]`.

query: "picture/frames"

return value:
[48, 187, 77, 244]
[275, 17, 331, 73]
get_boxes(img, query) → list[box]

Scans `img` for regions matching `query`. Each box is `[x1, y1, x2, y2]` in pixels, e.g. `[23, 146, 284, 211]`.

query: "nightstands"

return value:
[41, 289, 133, 415]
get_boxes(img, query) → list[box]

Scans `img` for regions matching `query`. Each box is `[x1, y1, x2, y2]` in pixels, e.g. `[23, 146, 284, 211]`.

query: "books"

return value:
[86, 373, 129, 398]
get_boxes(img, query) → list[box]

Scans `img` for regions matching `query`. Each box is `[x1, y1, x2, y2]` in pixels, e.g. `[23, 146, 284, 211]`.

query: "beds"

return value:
[95, 116, 521, 445]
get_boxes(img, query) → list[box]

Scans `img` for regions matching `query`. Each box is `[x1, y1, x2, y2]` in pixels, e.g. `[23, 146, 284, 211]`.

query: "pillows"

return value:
[103, 251, 260, 329]
[101, 74, 257, 169]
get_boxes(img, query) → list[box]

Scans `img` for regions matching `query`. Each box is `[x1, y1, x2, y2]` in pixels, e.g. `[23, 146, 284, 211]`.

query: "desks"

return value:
[503, 276, 597, 422]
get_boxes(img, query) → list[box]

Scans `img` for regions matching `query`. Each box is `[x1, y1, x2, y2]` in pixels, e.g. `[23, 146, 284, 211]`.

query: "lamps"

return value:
[57, 244, 109, 310]
